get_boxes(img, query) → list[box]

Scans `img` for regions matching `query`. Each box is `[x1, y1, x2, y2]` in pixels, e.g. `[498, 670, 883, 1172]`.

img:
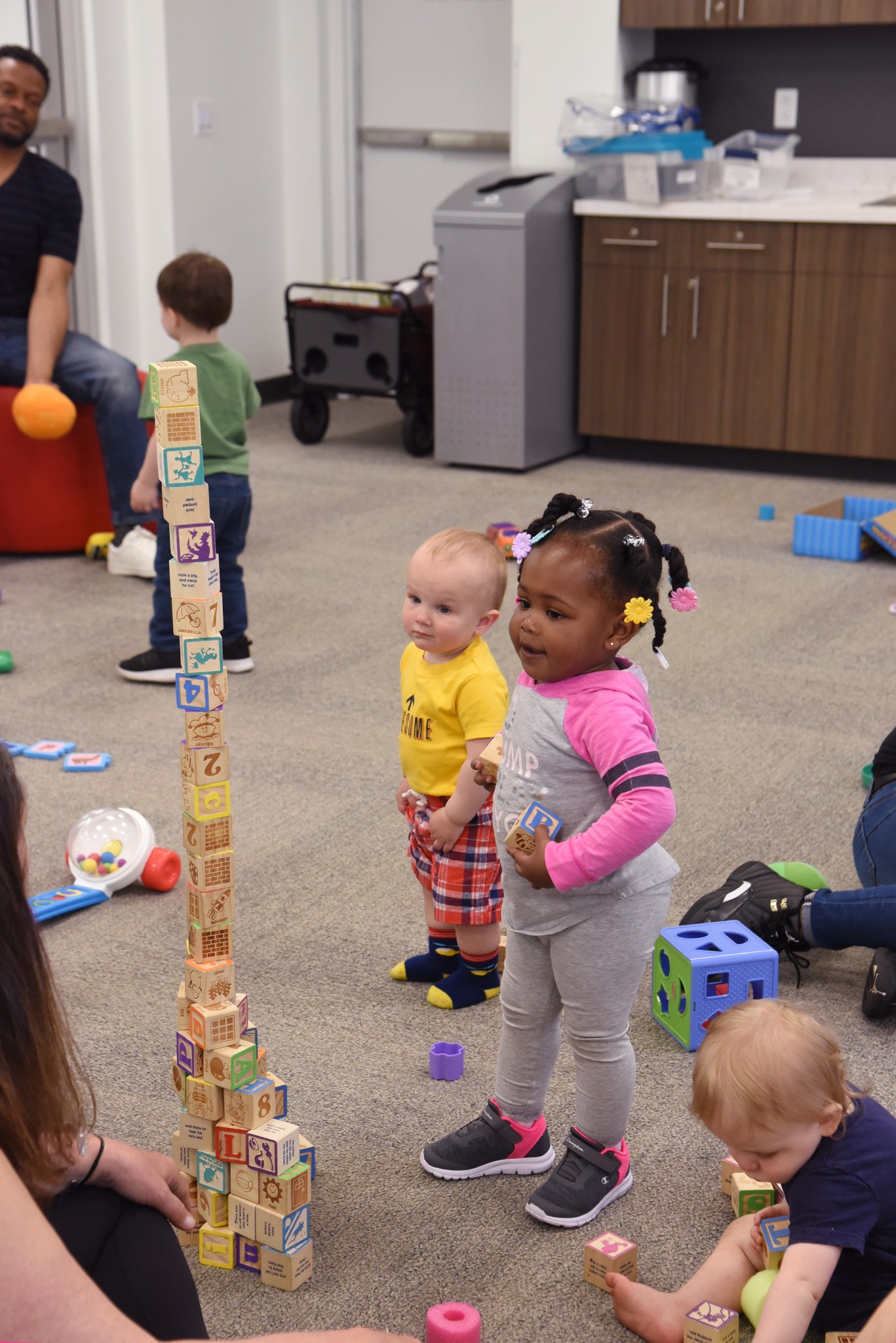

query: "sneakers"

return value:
[862, 945, 896, 1018]
[116, 648, 185, 683]
[420, 1097, 555, 1180]
[679, 860, 813, 990]
[108, 524, 159, 578]
[221, 636, 255, 674]
[525, 1126, 633, 1228]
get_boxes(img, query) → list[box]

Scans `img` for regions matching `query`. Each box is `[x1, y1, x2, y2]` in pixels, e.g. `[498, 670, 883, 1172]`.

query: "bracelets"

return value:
[72, 1131, 105, 1187]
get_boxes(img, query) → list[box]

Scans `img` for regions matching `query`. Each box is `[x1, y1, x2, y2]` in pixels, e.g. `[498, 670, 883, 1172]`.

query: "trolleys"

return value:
[284, 259, 438, 461]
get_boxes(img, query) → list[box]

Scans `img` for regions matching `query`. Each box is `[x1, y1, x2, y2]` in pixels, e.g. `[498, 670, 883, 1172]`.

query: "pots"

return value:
[635, 53, 696, 107]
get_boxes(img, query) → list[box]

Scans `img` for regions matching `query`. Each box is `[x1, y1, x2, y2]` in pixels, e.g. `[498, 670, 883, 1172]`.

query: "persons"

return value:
[418, 494, 701, 1234]
[0, 743, 424, 1343]
[382, 524, 515, 1006]
[109, 248, 261, 680]
[602, 997, 895, 1342]
[0, 44, 169, 578]
[682, 721, 896, 1017]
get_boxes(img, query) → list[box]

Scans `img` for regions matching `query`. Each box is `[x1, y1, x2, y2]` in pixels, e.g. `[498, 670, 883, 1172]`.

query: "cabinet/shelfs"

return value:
[840, 0, 896, 26]
[577, 216, 795, 470]
[787, 222, 896, 486]
[620, 0, 840, 30]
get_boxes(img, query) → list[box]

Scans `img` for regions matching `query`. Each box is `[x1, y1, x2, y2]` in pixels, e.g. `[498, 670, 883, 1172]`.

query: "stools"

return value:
[0, 370, 162, 552]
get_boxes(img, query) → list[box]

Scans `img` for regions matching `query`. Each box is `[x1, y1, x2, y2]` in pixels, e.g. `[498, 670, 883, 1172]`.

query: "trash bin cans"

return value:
[428, 164, 594, 475]
